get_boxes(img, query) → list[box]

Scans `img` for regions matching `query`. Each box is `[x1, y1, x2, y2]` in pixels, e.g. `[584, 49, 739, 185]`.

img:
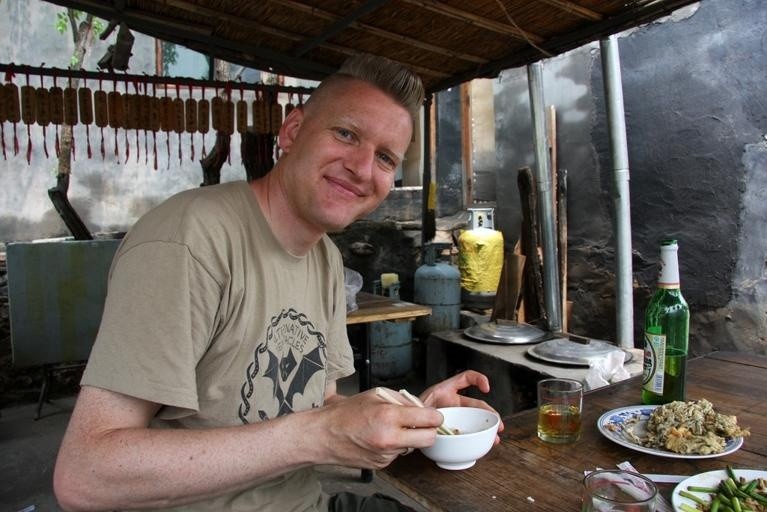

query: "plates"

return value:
[595, 404, 744, 457]
[670, 468, 766, 512]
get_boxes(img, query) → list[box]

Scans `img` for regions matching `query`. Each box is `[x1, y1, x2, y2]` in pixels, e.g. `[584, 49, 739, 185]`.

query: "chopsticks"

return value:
[375, 387, 453, 434]
[580, 460, 691, 511]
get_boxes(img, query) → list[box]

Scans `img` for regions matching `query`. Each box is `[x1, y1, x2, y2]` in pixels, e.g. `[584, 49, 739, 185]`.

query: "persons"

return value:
[49, 49, 506, 512]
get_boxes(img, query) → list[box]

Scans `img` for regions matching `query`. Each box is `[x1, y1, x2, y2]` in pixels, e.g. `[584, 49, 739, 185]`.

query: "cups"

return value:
[536, 378, 581, 442]
[579, 470, 658, 512]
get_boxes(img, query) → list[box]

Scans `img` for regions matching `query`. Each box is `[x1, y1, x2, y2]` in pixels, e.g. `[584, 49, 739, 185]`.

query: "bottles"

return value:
[640, 240, 691, 404]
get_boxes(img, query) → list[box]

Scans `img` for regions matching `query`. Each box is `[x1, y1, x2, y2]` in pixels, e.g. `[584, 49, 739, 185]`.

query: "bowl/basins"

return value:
[417, 407, 500, 471]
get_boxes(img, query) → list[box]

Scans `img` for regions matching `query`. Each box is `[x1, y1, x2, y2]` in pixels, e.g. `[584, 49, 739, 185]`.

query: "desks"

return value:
[370, 347, 766, 512]
[333, 289, 434, 397]
